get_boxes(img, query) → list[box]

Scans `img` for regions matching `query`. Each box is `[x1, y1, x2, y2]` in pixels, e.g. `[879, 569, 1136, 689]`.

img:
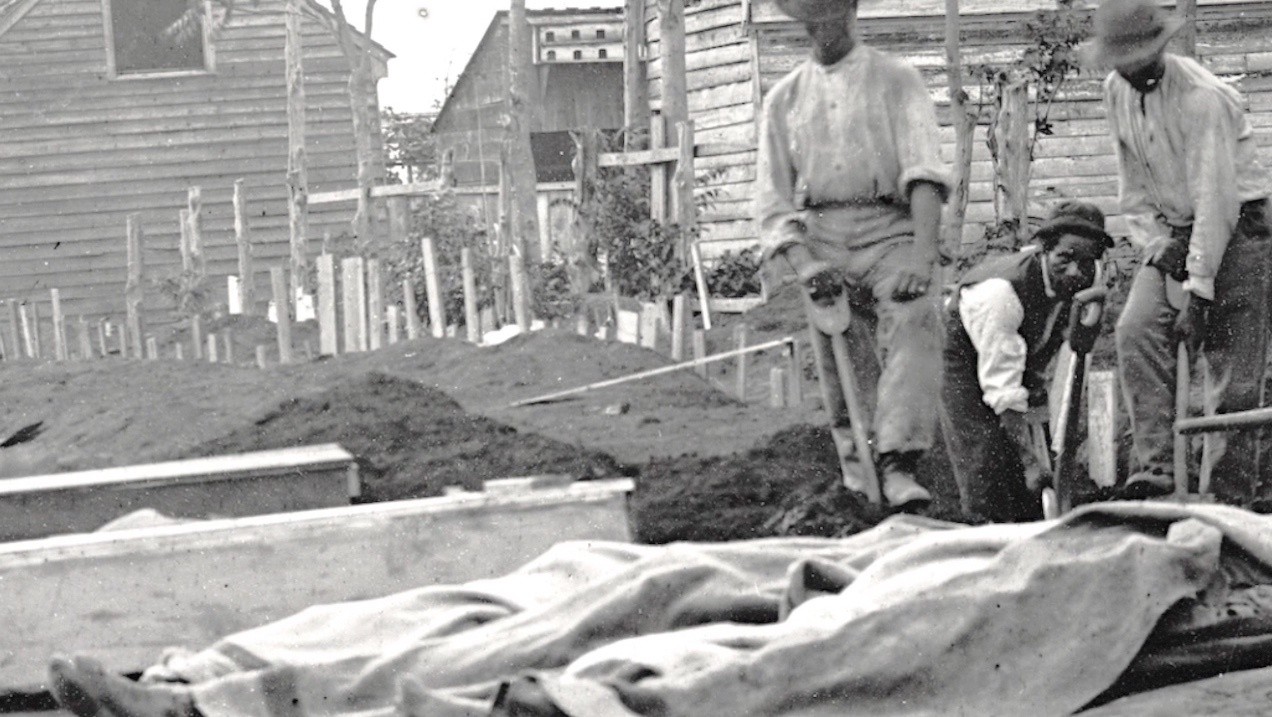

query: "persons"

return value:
[1095, 0, 1272, 498]
[934, 200, 1115, 528]
[754, 0, 952, 511]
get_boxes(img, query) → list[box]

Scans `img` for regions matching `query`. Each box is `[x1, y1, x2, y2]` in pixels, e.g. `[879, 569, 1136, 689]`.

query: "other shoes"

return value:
[878, 458, 930, 508]
[1127, 470, 1199, 496]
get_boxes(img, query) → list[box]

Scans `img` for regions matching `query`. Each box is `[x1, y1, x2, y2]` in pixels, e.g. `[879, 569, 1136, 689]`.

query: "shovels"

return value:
[1041, 298, 1104, 523]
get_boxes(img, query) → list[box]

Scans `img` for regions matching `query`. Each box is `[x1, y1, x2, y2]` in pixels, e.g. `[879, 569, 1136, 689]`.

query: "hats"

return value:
[777, 0, 855, 21]
[1027, 200, 1114, 249]
[1076, 0, 1187, 68]
